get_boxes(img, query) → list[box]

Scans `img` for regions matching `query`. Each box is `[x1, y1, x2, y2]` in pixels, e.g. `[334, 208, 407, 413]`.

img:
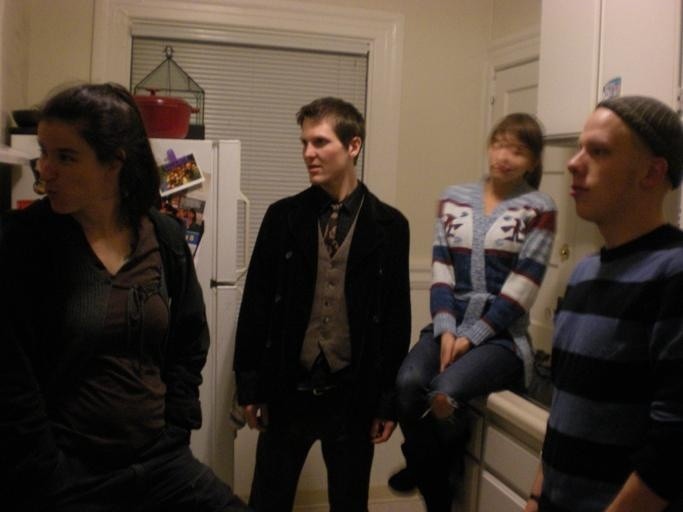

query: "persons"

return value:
[387, 112, 556, 512]
[1, 82, 253, 511]
[232, 97, 408, 511]
[522, 95, 682, 512]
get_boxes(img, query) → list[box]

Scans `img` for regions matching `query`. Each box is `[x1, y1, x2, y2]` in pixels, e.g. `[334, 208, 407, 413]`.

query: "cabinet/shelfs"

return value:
[537, 2, 679, 142]
[476, 416, 543, 512]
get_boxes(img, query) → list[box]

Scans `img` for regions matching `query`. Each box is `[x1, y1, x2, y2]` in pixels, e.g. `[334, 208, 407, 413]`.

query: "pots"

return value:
[128, 86, 200, 139]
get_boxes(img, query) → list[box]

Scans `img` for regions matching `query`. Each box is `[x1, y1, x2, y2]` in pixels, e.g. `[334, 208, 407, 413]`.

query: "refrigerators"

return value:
[9, 130, 253, 498]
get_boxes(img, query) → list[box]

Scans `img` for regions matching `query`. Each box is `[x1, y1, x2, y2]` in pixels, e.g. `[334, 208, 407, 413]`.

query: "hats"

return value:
[596, 95, 682, 184]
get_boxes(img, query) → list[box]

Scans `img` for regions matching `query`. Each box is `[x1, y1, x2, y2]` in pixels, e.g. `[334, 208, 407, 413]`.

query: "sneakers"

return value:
[388, 467, 418, 493]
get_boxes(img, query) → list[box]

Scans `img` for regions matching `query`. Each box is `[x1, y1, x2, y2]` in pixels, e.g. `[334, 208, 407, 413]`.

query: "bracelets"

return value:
[529, 492, 539, 502]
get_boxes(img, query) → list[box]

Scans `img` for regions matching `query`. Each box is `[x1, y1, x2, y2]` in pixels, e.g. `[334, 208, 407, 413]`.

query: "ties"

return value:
[322, 203, 341, 252]
